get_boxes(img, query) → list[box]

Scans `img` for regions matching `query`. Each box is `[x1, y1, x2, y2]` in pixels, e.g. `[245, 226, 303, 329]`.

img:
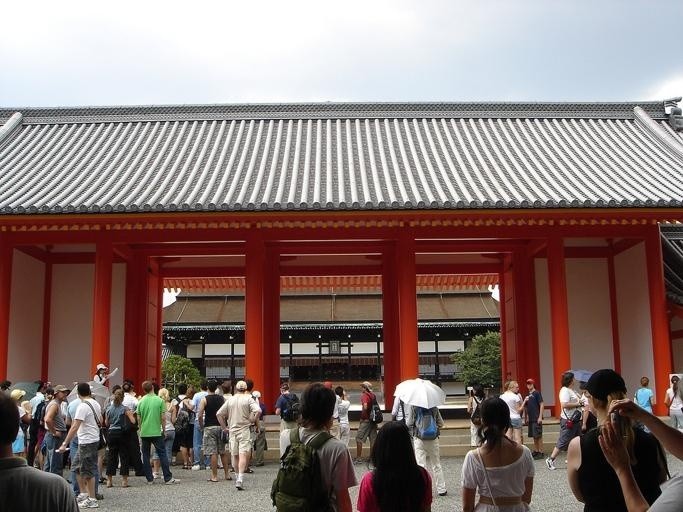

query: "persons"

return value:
[198, 380, 232, 482]
[152, 382, 160, 396]
[122, 383, 143, 476]
[35, 388, 54, 456]
[123, 379, 142, 462]
[27, 381, 48, 467]
[93, 364, 118, 386]
[275, 384, 302, 464]
[216, 381, 262, 490]
[467, 390, 489, 446]
[0, 391, 80, 512]
[599, 398, 682, 512]
[353, 381, 383, 464]
[183, 381, 211, 470]
[664, 376, 683, 430]
[43, 385, 71, 477]
[567, 369, 666, 512]
[505, 382, 523, 403]
[34, 389, 60, 446]
[336, 386, 350, 446]
[324, 382, 342, 440]
[105, 389, 135, 488]
[392, 397, 412, 421]
[58, 383, 103, 508]
[633, 377, 656, 433]
[524, 379, 546, 460]
[406, 406, 448, 495]
[357, 421, 433, 512]
[250, 391, 268, 467]
[218, 381, 232, 468]
[462, 396, 535, 511]
[546, 373, 583, 470]
[89, 392, 108, 483]
[66, 397, 104, 500]
[499, 381, 523, 445]
[271, 383, 359, 512]
[104, 385, 121, 413]
[171, 383, 196, 466]
[153, 388, 176, 479]
[60, 398, 67, 469]
[579, 381, 597, 434]
[178, 387, 196, 468]
[11, 389, 32, 458]
[137, 380, 181, 484]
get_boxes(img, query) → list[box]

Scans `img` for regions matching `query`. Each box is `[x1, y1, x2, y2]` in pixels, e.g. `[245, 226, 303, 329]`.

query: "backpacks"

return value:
[271, 428, 336, 512]
[471, 397, 486, 426]
[363, 392, 383, 425]
[281, 393, 303, 423]
[178, 399, 202, 428]
[176, 394, 190, 417]
[414, 407, 440, 439]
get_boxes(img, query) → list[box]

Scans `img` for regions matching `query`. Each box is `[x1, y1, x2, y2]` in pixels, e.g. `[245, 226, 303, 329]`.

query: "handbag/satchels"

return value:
[560, 409, 582, 430]
[120, 405, 139, 432]
[80, 400, 109, 449]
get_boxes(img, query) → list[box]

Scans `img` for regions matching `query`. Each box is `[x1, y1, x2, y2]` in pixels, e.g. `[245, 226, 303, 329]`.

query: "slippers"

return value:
[207, 477, 218, 482]
[224, 476, 232, 480]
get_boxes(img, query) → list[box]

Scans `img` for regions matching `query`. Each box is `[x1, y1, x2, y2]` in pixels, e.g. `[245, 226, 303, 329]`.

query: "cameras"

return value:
[526, 394, 534, 398]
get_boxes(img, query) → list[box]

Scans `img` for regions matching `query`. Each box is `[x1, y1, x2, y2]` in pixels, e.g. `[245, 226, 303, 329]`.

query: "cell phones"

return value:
[467, 387, 473, 390]
[610, 411, 628, 438]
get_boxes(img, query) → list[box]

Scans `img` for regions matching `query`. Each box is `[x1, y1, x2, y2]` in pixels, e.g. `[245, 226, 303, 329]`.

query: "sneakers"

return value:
[366, 457, 374, 461]
[122, 477, 130, 487]
[166, 479, 180, 484]
[205, 466, 210, 470]
[531, 451, 538, 456]
[353, 457, 363, 464]
[191, 465, 201, 470]
[107, 481, 113, 487]
[153, 473, 159, 479]
[170, 461, 176, 466]
[78, 499, 99, 507]
[135, 471, 145, 477]
[439, 490, 447, 496]
[147, 480, 154, 485]
[99, 478, 104, 482]
[161, 473, 165, 479]
[545, 457, 556, 470]
[256, 461, 265, 467]
[182, 465, 188, 469]
[245, 468, 253, 473]
[76, 495, 89, 501]
[534, 453, 544, 460]
[235, 481, 247, 490]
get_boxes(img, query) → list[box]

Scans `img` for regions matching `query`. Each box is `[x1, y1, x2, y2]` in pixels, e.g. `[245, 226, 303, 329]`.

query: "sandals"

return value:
[230, 467, 236, 472]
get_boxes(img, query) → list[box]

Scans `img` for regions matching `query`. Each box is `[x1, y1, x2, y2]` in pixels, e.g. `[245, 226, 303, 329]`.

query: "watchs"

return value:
[63, 442, 68, 446]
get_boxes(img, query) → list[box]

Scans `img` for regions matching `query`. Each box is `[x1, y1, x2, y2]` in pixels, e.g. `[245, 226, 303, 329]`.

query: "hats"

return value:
[361, 381, 373, 392]
[10, 389, 26, 401]
[236, 381, 247, 391]
[252, 391, 261, 398]
[586, 369, 627, 400]
[96, 364, 108, 373]
[54, 385, 70, 394]
[526, 378, 535, 384]
[280, 384, 290, 390]
[324, 382, 333, 388]
